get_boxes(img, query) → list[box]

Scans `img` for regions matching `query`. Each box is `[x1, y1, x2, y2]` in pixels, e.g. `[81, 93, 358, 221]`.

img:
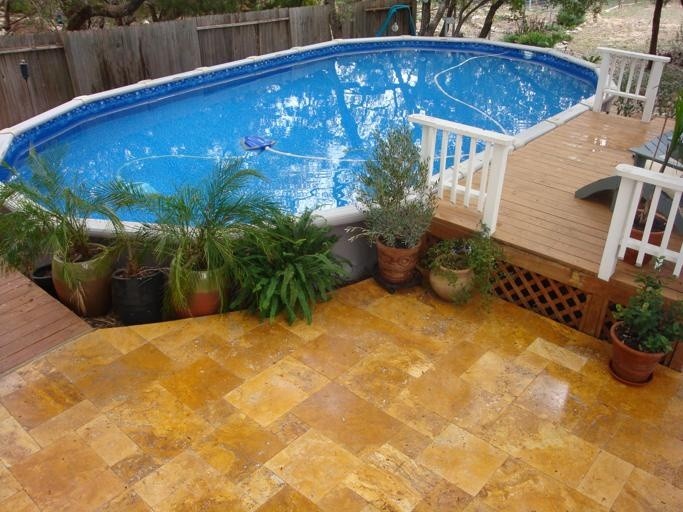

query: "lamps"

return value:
[390, 12, 400, 32]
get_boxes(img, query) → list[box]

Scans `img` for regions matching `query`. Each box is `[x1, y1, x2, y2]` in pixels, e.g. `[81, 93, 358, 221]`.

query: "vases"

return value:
[28, 261, 55, 295]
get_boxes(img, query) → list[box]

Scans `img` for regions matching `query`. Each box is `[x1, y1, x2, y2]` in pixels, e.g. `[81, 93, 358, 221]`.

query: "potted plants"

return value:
[69, 190, 164, 324]
[343, 130, 442, 284]
[226, 207, 351, 326]
[95, 158, 296, 319]
[608, 256, 683, 386]
[0, 132, 117, 318]
[423, 221, 506, 303]
[623, 80, 683, 265]
